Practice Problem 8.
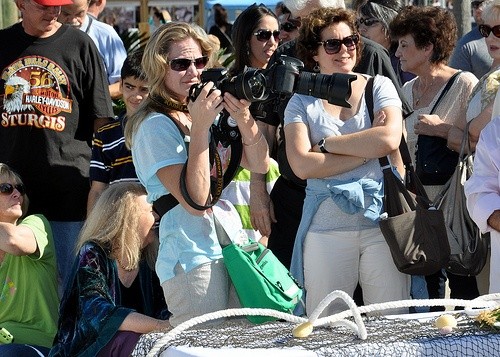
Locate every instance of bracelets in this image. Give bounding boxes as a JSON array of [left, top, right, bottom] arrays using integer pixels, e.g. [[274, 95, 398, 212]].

[[364, 158, 367, 165], [242, 133, 264, 148]]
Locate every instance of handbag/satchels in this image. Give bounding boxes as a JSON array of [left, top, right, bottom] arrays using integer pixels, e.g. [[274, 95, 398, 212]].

[[433, 160, 489, 276], [379, 171, 452, 275], [415, 70, 464, 186], [221, 242, 304, 325]]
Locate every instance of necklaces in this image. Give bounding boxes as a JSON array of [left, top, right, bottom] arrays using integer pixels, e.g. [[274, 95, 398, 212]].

[[415, 68, 441, 106], [117, 253, 136, 272]]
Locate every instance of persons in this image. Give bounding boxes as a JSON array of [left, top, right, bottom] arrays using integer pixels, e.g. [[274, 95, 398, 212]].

[[0, 162, 60, 357], [47, 182, 172, 357], [284, 8, 412, 320], [0, 0, 500, 313], [125, 23, 270, 327], [386, 5, 480, 312]]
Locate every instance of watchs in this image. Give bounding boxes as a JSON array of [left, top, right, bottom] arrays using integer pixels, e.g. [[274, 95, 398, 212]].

[[318, 137, 328, 153]]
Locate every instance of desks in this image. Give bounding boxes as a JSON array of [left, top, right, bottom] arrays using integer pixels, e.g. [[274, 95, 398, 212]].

[[130, 308, 500, 357]]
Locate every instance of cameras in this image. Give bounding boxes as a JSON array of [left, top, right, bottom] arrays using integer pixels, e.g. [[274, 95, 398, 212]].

[[194, 66, 268, 103], [267, 53, 358, 108]]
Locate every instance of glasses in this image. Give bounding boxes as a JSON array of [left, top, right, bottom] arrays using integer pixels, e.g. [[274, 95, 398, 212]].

[[287, 13, 301, 28], [471, 0, 484, 7], [169, 56, 210, 72], [0, 183, 25, 196], [254, 29, 280, 42], [280, 21, 294, 33], [320, 35, 359, 54], [478, 23, 500, 38], [360, 17, 380, 27]]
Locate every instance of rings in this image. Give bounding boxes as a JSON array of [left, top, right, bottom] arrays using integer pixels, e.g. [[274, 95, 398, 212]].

[[234, 109, 239, 114], [417, 119, 420, 125]]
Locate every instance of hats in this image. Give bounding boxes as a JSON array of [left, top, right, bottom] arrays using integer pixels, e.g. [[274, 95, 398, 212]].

[[32, 0, 74, 5], [154, 7, 171, 24]]
